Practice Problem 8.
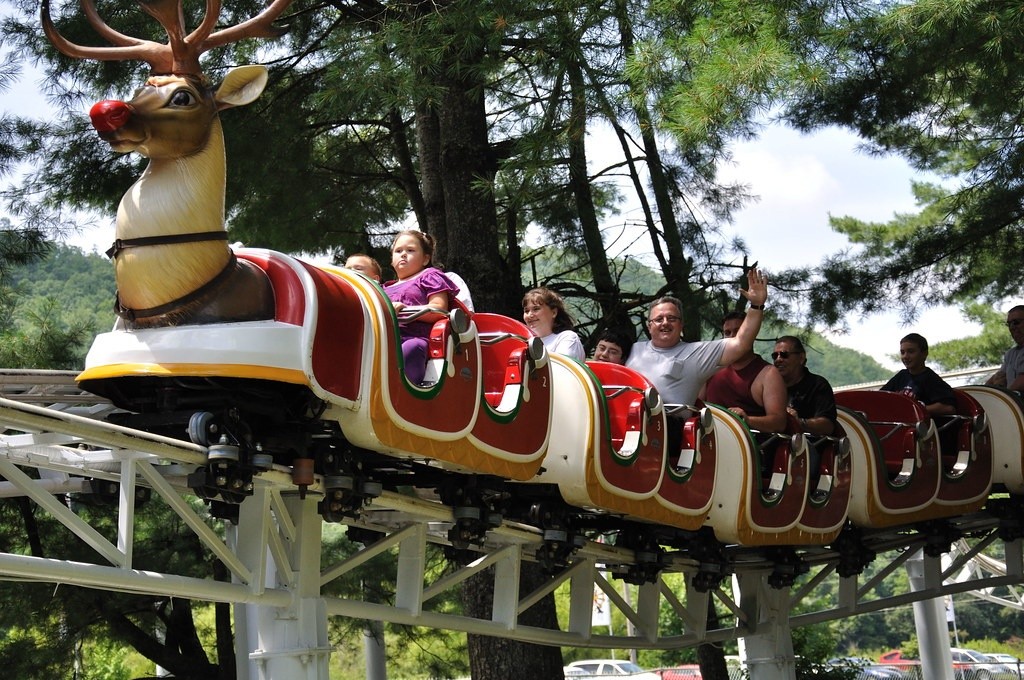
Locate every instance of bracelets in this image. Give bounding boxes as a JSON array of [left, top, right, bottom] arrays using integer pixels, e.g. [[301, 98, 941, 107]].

[[751, 304, 765, 310], [799, 418, 806, 425]]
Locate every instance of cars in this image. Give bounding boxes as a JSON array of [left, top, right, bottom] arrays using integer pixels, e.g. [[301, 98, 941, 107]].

[[564, 658, 661, 680], [655, 634, 1024, 680]]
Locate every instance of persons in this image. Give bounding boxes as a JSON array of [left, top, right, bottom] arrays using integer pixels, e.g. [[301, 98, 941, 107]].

[[985, 305, 1024, 406], [522, 288, 586, 363], [697, 310, 788, 476], [594, 329, 632, 364], [771, 335, 837, 480], [345, 253, 381, 282], [625, 268, 768, 454], [879, 333, 957, 456], [379, 230, 459, 385]]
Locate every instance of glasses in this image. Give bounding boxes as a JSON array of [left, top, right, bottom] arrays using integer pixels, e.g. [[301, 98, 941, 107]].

[[1006, 318, 1024, 326], [771, 350, 800, 359], [649, 314, 682, 323]]
